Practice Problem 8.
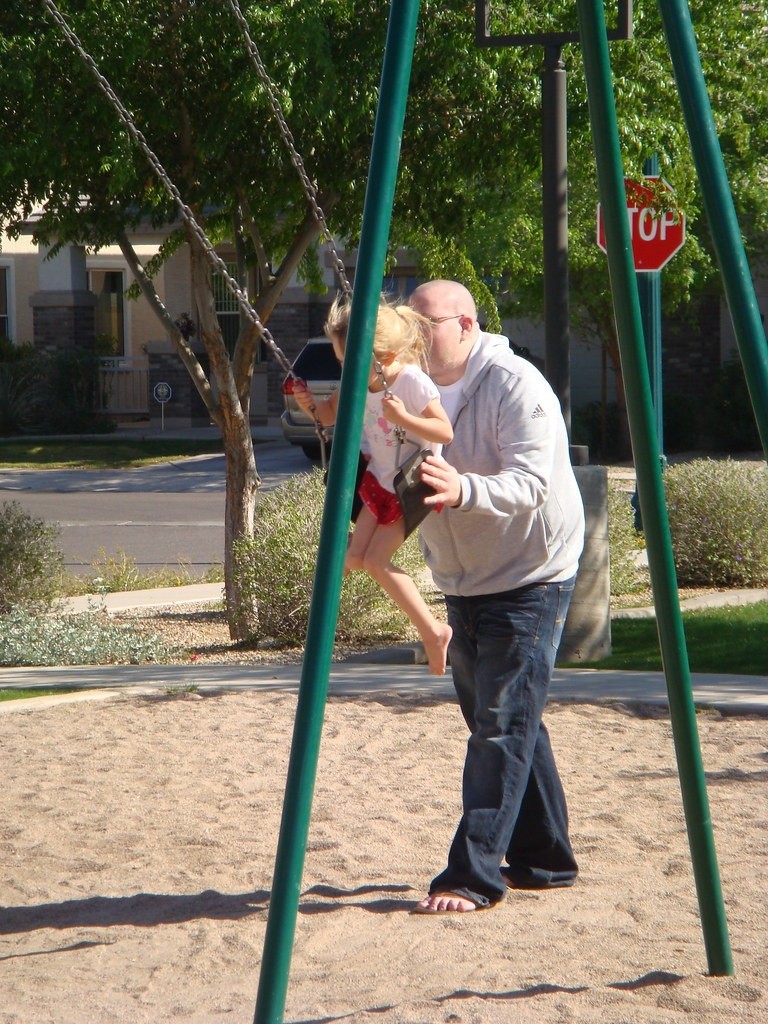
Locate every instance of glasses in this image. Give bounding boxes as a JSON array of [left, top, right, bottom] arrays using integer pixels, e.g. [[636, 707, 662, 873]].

[[418, 313, 464, 326]]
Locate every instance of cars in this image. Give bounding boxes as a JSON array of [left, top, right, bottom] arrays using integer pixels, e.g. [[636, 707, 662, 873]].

[[281, 335, 530, 459]]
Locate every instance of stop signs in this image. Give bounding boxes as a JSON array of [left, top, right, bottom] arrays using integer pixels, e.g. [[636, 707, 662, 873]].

[[595, 176, 685, 272]]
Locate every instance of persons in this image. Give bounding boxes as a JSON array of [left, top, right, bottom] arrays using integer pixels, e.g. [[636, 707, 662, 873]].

[[390, 276, 591, 916], [285, 297, 462, 677]]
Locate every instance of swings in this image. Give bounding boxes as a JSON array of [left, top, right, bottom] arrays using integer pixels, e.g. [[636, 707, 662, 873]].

[[39, 1, 440, 541]]
